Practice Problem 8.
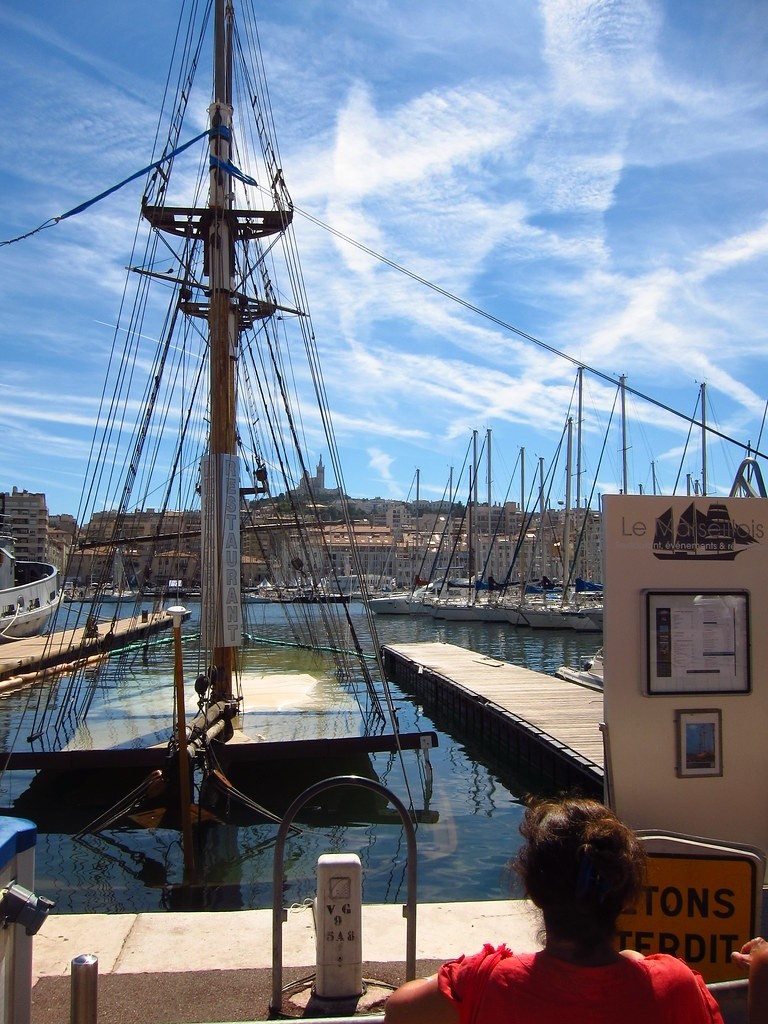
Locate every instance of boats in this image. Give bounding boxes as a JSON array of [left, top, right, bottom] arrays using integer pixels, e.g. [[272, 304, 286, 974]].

[[0, 508, 66, 646], [555, 647, 605, 693]]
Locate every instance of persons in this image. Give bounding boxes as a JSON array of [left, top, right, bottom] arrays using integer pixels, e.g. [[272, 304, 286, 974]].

[[80, 587, 84, 597], [515, 586, 519, 595], [384, 793, 768, 1024], [568, 588, 572, 601]]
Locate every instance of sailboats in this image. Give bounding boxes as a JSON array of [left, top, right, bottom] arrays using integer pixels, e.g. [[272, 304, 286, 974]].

[[57, 364, 768, 635]]
